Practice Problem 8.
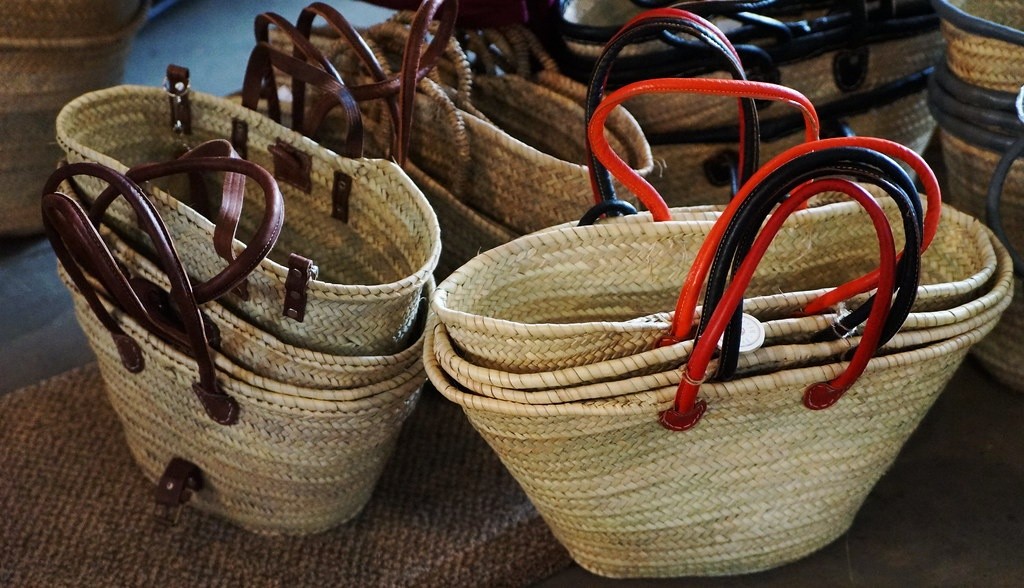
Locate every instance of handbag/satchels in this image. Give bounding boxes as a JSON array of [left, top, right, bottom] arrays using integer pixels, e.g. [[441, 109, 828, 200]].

[[38, 1, 1024, 579]]
